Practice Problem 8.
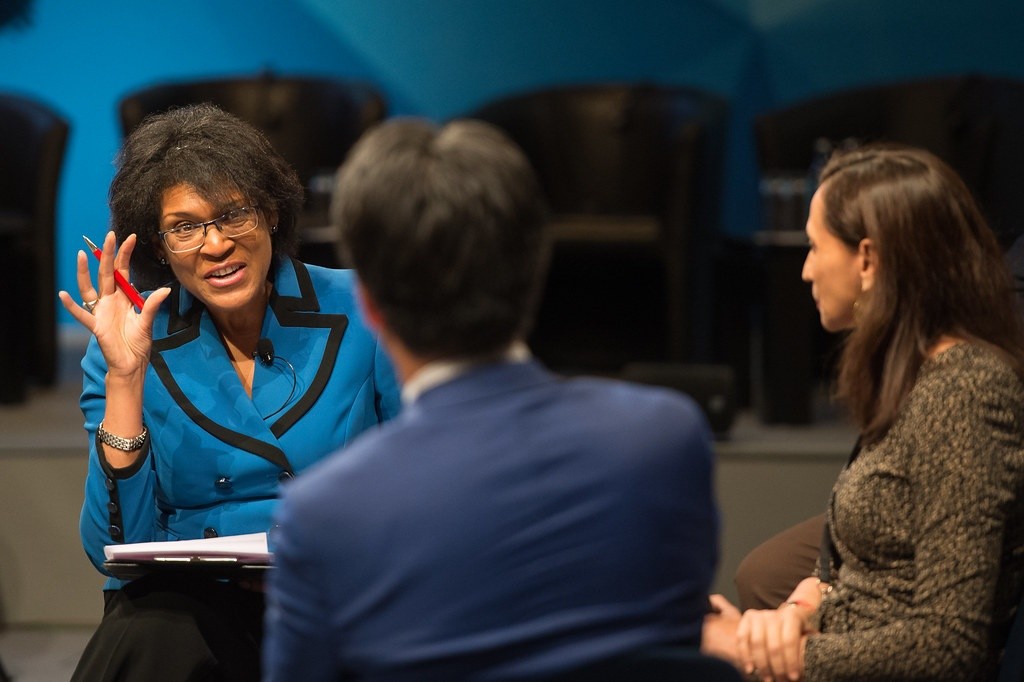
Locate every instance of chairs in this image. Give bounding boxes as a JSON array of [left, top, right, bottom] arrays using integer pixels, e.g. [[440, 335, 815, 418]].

[[0, 91, 71, 407], [751, 68, 1024, 420], [114, 72, 394, 269], [446, 75, 727, 376]]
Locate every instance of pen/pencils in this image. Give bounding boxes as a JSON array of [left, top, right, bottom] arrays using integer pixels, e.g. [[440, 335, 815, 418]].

[[82, 235, 145, 311]]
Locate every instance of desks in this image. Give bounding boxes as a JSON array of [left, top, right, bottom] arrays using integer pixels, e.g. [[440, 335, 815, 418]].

[[297, 215, 663, 337]]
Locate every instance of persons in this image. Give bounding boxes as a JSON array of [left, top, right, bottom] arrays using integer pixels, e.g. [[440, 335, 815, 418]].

[[264, 114, 722, 682], [696, 143, 1024, 682], [59, 107, 405, 681]]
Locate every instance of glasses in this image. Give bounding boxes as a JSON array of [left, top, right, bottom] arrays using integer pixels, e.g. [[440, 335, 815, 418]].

[[157, 202, 258, 253]]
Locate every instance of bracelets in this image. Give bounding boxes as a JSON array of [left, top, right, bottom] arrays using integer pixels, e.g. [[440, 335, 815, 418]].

[[99, 419, 147, 451]]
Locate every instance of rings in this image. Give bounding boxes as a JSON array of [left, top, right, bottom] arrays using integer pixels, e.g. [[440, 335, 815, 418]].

[[83, 301, 98, 310]]
